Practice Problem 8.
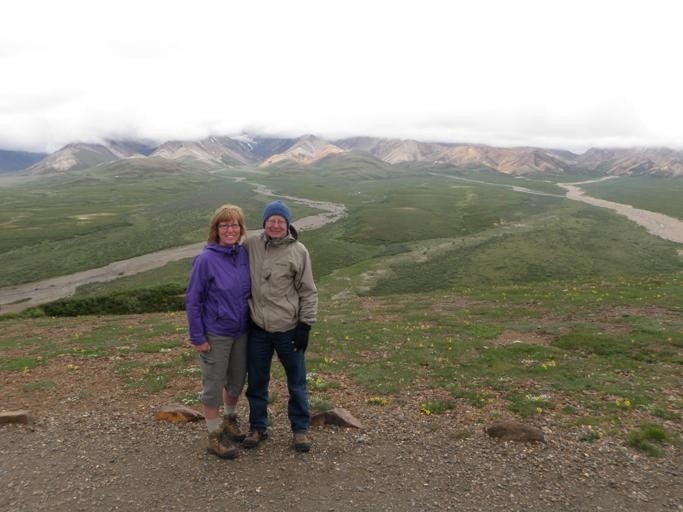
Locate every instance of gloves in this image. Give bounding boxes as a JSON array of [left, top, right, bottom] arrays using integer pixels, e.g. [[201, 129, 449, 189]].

[[291, 321, 311, 353]]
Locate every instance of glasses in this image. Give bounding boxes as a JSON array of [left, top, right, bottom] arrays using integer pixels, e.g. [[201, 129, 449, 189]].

[[265, 219, 288, 224], [216, 224, 239, 229]]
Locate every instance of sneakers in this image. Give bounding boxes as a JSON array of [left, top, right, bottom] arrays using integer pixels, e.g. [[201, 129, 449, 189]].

[[206, 430, 238, 458], [292, 433, 310, 451], [222, 413, 245, 441], [242, 431, 267, 447]]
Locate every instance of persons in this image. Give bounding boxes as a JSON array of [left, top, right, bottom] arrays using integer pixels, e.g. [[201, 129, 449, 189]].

[[185, 204, 252, 460], [240, 199, 318, 454]]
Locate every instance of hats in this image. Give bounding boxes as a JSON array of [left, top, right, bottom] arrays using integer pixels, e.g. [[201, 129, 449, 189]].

[[262, 200, 291, 228]]
